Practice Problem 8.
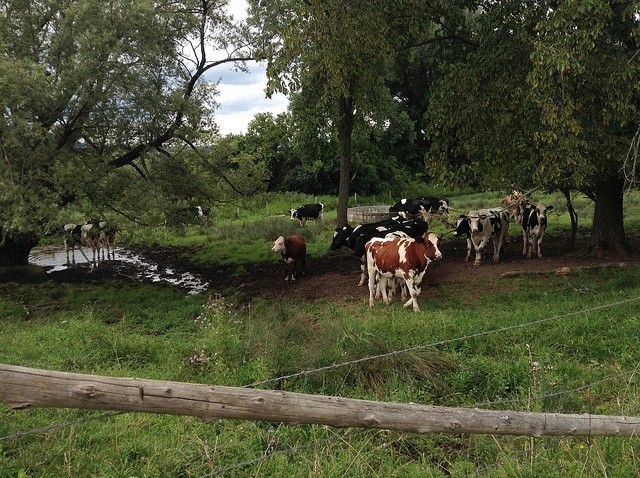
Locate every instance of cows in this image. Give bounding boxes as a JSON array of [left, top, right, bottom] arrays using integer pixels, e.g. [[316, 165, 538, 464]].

[[522, 203, 553, 259], [271, 235, 306, 280], [288, 203, 324, 226], [354, 220, 428, 287], [389, 198, 432, 222], [364, 232, 443, 312], [84, 220, 116, 260], [330, 224, 355, 251], [417, 197, 449, 216], [453, 214, 471, 261], [504, 190, 531, 225], [347, 220, 409, 246], [468, 208, 510, 265], [62, 224, 104, 264], [185, 206, 211, 225]]
